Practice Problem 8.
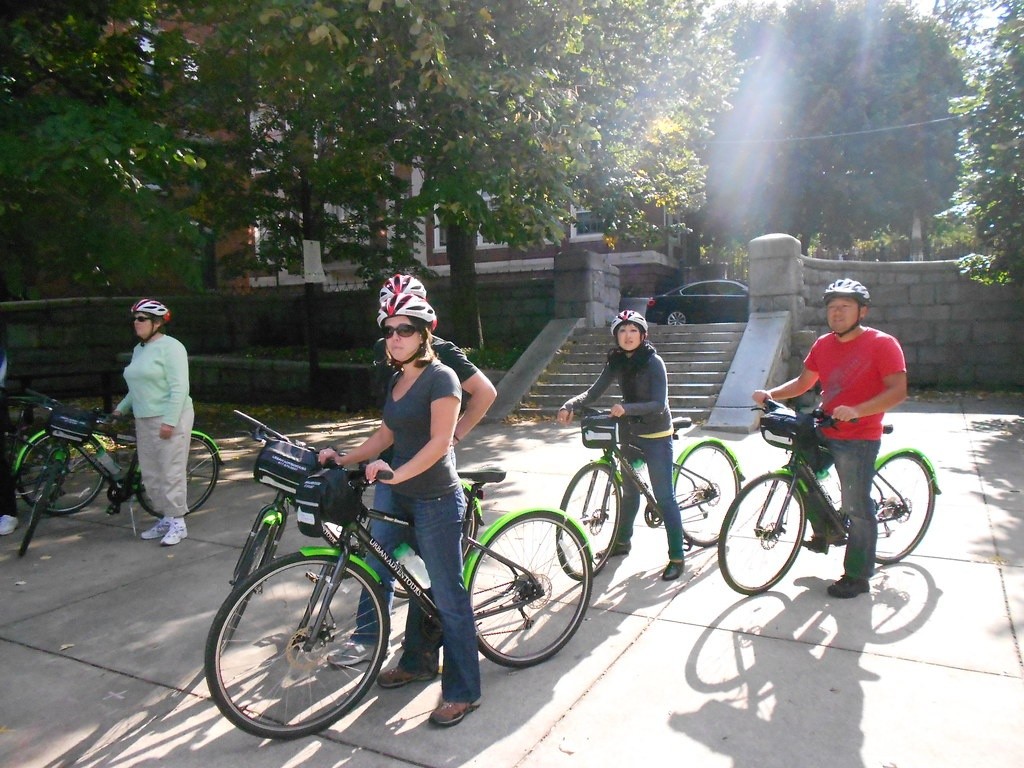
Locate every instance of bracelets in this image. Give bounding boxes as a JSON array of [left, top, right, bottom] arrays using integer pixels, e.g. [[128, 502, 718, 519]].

[[454, 435, 460, 442]]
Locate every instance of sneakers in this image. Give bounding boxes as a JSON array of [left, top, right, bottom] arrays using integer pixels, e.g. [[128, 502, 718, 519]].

[[325, 641, 393, 666], [160, 520, 188, 546], [428, 695, 481, 727], [376, 664, 437, 688], [0, 514, 20, 537], [140, 516, 173, 540]]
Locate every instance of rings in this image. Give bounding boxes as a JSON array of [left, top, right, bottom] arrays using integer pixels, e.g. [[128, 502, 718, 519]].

[[560, 418, 563, 420], [324, 452, 326, 454]]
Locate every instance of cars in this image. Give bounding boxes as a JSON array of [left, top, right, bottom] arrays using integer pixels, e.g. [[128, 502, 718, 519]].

[[644, 280, 750, 325]]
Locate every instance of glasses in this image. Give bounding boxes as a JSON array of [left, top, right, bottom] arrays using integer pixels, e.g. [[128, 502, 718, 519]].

[[130, 316, 153, 321], [380, 323, 419, 339]]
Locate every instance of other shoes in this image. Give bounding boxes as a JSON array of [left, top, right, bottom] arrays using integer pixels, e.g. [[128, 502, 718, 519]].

[[826, 576, 870, 599], [809, 536, 847, 551], [594, 541, 631, 558], [661, 558, 685, 582]]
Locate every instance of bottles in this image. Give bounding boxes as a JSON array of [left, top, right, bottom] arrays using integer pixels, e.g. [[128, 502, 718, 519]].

[[95, 447, 122, 474], [632, 458, 653, 489], [393, 543, 433, 590], [817, 469, 842, 503]]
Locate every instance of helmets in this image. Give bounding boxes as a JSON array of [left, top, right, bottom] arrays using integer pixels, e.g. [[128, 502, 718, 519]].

[[610, 309, 649, 335], [819, 278, 872, 309], [129, 298, 172, 323], [376, 273, 427, 301], [375, 292, 437, 334]]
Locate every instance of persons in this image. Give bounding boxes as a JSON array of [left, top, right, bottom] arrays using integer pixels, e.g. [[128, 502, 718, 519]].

[[557, 310, 685, 581], [752, 278, 907, 598], [0, 347, 19, 535], [318, 294, 481, 726], [100, 299, 195, 545], [326, 274, 498, 666]]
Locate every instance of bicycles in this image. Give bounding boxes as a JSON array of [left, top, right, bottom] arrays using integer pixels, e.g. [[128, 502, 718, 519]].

[[556, 407, 746, 581], [6, 389, 225, 557], [205, 411, 593, 739], [719, 399, 942, 596]]
